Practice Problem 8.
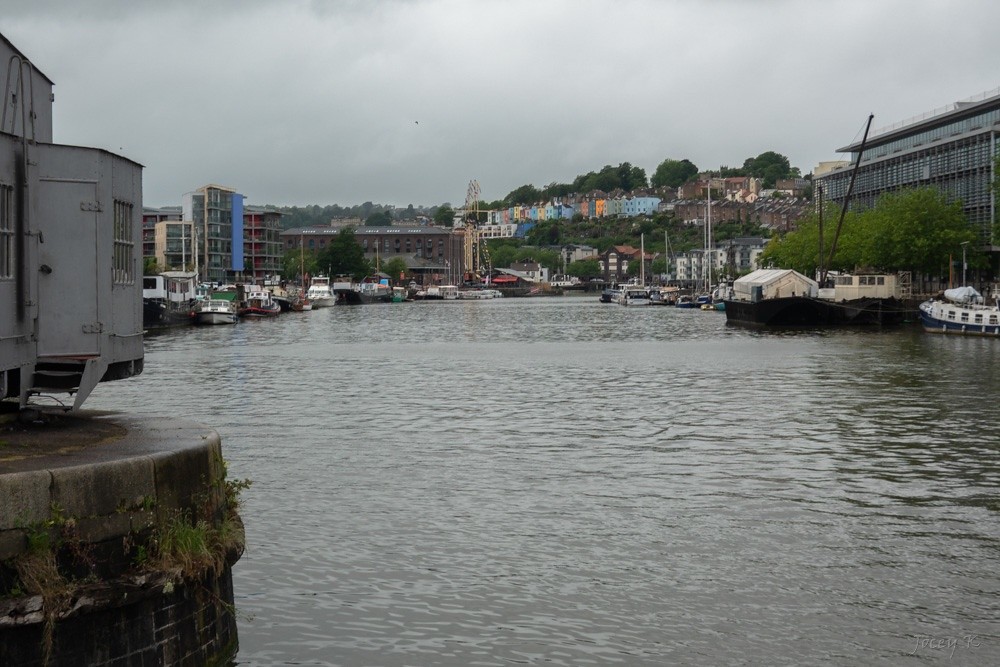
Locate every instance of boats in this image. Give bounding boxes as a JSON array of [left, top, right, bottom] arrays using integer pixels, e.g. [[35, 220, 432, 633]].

[[599, 184, 733, 310], [142, 270, 203, 329], [918, 253, 1000, 337], [196, 299, 238, 325], [242, 291, 281, 317], [334, 281, 392, 304], [415, 285, 504, 301], [391, 286, 409, 302], [307, 275, 337, 306], [262, 285, 291, 313]]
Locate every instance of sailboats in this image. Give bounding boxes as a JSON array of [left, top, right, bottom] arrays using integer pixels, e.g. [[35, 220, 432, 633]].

[[290, 230, 315, 311]]
